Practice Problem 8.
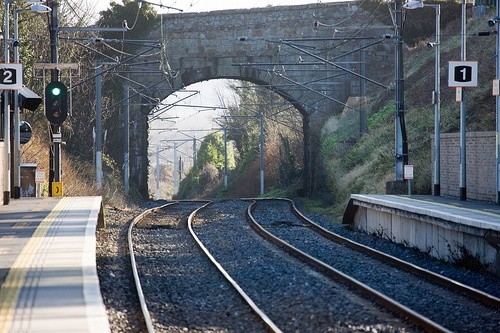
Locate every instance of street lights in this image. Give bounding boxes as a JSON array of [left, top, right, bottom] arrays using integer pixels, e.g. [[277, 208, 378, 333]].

[[402, 0, 441, 196], [14, 5, 52, 199]]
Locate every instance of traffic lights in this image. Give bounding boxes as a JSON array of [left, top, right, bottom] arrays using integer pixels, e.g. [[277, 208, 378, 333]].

[[45, 82, 68, 125]]
